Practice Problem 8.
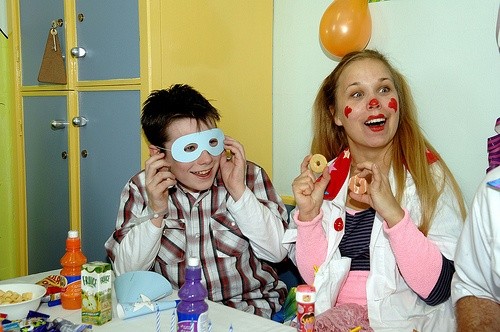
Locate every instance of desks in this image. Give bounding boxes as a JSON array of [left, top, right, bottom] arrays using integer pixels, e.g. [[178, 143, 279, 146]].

[[0, 268, 296, 332]]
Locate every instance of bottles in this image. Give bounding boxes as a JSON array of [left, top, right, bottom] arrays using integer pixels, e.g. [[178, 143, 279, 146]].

[[60, 231, 88, 309], [296, 285, 315, 332], [176, 257, 210, 332]]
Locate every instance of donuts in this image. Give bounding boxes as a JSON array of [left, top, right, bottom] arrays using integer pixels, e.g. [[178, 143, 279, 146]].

[[348, 175, 368, 195], [310, 153, 327, 173]]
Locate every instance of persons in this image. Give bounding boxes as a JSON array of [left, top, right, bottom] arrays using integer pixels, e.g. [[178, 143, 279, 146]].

[[104, 84, 288, 320], [283, 50, 469, 332], [450, 167, 500, 332]]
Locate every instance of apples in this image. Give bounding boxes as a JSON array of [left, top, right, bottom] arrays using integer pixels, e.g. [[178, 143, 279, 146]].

[[82, 296, 97, 310]]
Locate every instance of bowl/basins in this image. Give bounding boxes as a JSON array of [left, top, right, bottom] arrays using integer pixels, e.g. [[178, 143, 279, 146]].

[[0, 283, 47, 320]]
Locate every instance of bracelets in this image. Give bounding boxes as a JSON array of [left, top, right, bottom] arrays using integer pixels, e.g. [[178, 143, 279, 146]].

[[130, 207, 168, 223]]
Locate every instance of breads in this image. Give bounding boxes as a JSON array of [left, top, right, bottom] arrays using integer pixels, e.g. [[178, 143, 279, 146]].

[[0, 290, 33, 305]]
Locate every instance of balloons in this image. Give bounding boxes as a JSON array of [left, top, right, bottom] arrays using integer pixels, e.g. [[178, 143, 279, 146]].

[[319, 0, 372, 57]]
